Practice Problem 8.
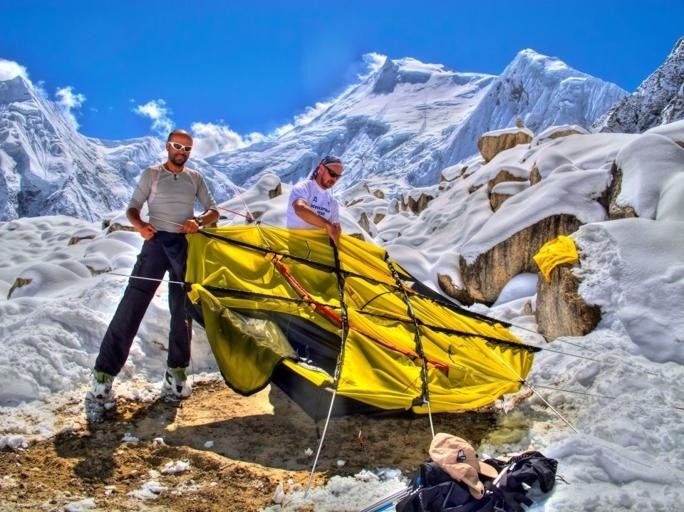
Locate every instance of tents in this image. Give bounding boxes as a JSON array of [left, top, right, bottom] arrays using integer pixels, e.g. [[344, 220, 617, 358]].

[[183, 210, 542, 426]]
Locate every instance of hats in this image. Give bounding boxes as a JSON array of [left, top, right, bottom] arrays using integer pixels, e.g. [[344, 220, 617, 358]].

[[429, 432, 499, 478]]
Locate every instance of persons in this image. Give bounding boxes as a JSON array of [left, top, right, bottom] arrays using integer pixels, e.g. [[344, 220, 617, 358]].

[[89, 128, 220, 400], [286, 156, 343, 247]]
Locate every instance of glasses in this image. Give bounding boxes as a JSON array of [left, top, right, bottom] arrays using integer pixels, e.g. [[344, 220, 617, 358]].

[[324, 164, 341, 180], [168, 141, 192, 152]]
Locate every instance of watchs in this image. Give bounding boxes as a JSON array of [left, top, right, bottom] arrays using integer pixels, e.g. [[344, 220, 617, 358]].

[[192, 213, 205, 228]]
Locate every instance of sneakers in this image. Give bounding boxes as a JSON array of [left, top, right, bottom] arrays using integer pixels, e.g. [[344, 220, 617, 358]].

[[164, 365, 192, 399], [92, 373, 112, 399]]
